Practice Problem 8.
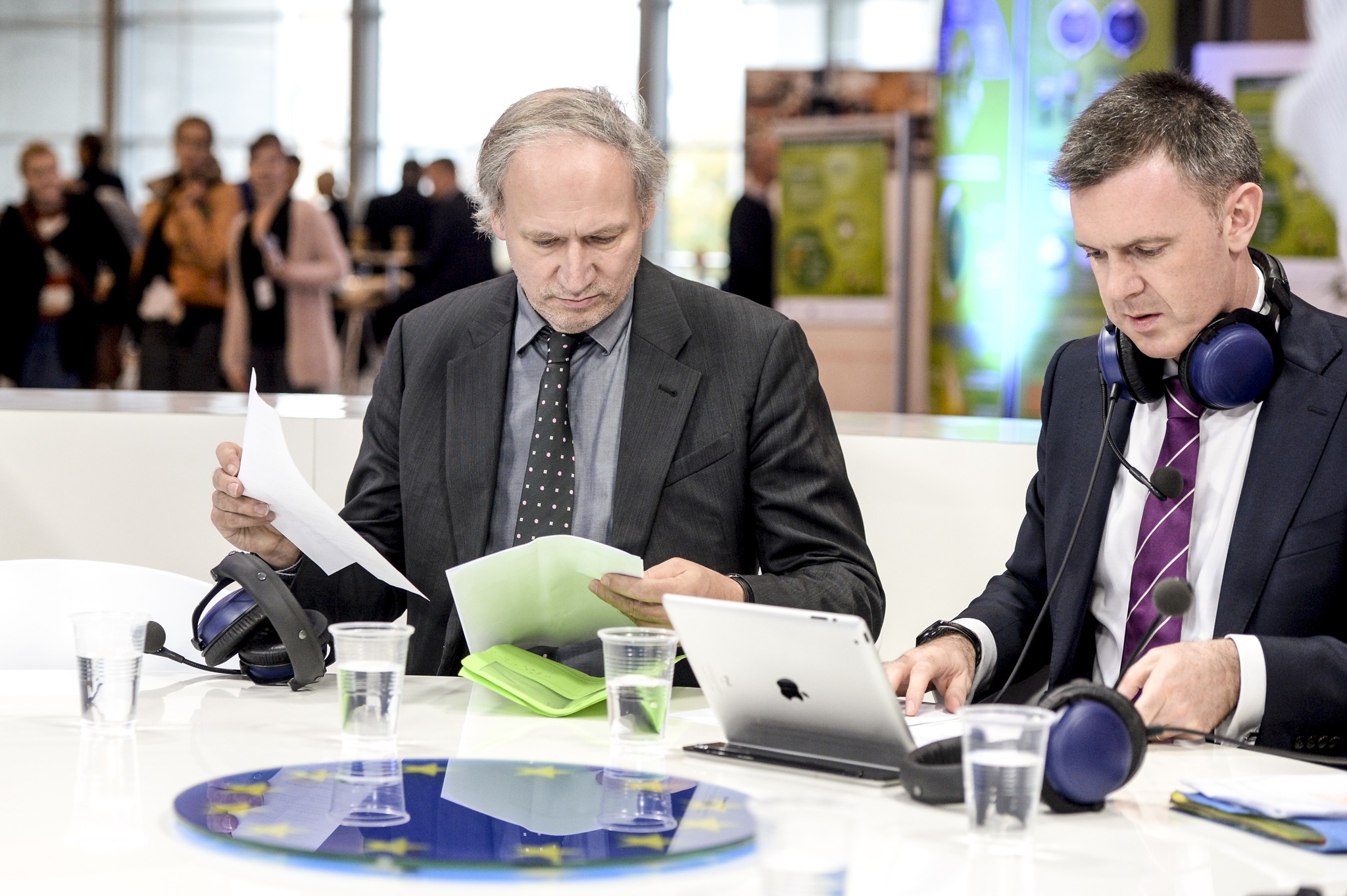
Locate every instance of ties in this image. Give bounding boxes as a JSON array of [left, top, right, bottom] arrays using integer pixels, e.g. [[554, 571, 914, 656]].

[[1116, 368, 1208, 743], [512, 326, 596, 660]]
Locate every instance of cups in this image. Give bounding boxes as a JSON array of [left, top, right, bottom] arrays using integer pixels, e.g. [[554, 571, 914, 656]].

[[326, 620, 416, 741], [69, 606, 150, 726], [596, 769, 679, 833], [598, 626, 679, 741], [334, 760, 410, 826], [957, 704, 1060, 835]]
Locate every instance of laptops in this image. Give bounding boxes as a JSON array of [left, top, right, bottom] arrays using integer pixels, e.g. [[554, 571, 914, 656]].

[[661, 593, 1027, 767]]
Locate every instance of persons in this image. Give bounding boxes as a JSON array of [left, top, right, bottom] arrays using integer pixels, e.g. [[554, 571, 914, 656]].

[[208, 86, 886, 687], [716, 130, 784, 310], [0, 115, 508, 397], [880, 62, 1347, 759]]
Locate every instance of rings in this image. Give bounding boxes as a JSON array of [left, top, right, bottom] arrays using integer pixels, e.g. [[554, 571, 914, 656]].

[[1153, 732, 1162, 743]]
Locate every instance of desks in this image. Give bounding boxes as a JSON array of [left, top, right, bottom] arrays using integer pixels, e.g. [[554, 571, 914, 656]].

[[0, 676, 1347, 896]]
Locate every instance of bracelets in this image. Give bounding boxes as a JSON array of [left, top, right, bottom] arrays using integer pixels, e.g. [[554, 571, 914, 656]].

[[916, 618, 982, 666], [722, 571, 757, 603]]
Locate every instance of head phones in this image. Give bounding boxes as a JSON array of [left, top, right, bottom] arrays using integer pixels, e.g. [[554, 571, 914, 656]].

[[187, 551, 335, 690], [898, 680, 1148, 815], [1100, 247, 1291, 409]]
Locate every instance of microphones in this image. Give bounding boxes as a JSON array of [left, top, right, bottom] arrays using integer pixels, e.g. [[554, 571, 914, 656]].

[[1114, 577, 1194, 693], [1103, 384, 1187, 500], [134, 620, 246, 676]]
